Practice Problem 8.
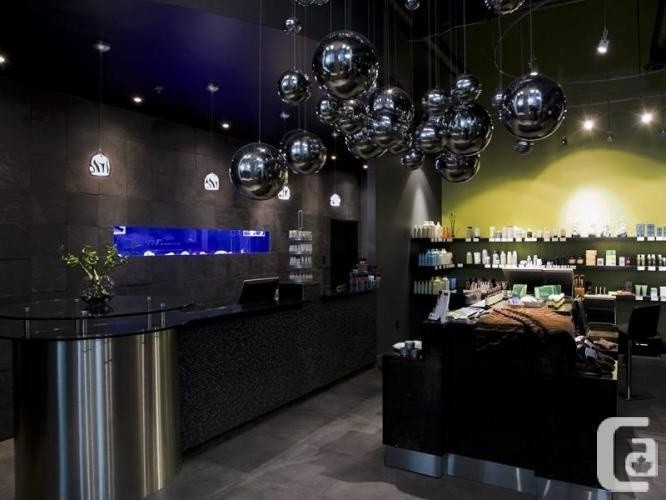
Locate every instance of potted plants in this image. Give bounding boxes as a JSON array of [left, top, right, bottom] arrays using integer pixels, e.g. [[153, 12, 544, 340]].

[[58, 242, 132, 309]]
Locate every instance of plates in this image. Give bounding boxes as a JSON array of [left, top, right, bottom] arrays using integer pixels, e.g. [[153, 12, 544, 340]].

[[393, 340, 423, 351]]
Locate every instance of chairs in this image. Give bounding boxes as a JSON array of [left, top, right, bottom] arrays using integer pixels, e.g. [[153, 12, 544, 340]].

[[571, 298, 621, 363], [613, 303, 661, 400]]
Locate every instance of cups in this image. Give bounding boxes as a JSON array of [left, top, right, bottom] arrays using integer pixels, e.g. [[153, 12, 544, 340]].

[[399, 341, 420, 360]]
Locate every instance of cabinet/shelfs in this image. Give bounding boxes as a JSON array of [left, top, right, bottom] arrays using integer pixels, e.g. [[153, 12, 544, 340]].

[[407, 234, 457, 329], [287, 209, 313, 281], [455, 237, 665, 307]]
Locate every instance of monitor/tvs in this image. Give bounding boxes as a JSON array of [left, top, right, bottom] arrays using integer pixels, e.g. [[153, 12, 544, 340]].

[[573, 294, 590, 338], [237, 277, 279, 305]]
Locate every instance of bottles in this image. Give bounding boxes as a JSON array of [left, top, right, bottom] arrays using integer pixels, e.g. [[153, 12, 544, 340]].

[[411, 219, 457, 296], [465, 248, 517, 265], [287, 229, 314, 282], [626, 257, 631, 265], [366, 274, 375, 289], [568, 255, 585, 265], [466, 225, 480, 239]]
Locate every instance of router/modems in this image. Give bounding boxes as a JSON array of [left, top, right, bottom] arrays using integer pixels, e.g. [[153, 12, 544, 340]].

[[584, 286, 616, 300]]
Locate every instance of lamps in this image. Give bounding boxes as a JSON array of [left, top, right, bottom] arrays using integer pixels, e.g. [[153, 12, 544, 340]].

[[87, 42, 115, 178], [202, 82, 223, 194]]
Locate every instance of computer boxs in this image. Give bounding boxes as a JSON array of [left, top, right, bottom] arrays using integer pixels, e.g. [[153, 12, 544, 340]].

[[583, 299, 616, 325]]
